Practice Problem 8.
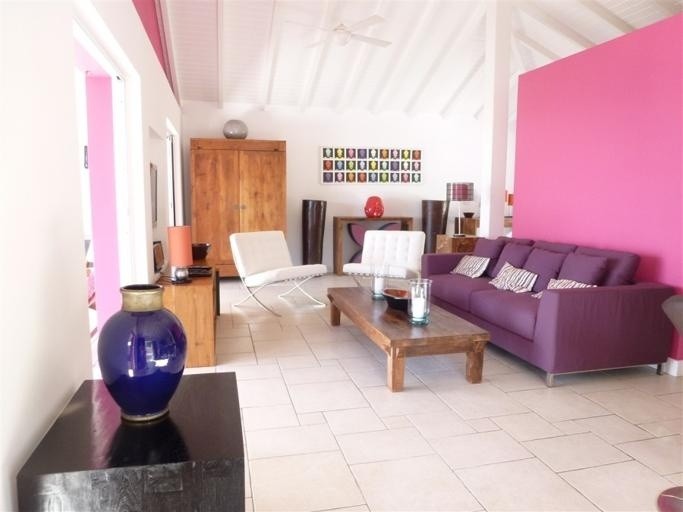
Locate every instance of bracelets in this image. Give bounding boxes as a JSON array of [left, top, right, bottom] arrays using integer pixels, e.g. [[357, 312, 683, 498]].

[[190, 241, 211, 261], [463, 211, 475, 218]]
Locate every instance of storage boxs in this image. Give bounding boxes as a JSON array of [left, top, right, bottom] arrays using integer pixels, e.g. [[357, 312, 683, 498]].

[[226, 228, 329, 313], [342, 229, 427, 289]]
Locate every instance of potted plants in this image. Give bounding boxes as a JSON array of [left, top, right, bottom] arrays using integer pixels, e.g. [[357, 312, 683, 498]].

[[148, 162, 158, 230], [152, 239, 165, 272]]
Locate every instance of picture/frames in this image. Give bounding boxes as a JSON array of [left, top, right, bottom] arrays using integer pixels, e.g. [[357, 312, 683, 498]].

[[17, 369, 246, 511], [153, 267, 220, 367], [333, 215, 413, 275]]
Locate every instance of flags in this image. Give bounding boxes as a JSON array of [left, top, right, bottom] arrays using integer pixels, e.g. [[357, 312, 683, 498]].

[[95, 284, 188, 427]]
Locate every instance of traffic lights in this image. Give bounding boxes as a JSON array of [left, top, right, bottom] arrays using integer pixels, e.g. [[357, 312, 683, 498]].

[[491, 241, 534, 284], [556, 252, 608, 289], [519, 246, 565, 293], [471, 236, 504, 277]]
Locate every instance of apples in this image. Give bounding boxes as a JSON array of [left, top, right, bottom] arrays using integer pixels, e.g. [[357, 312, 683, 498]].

[[412, 297, 424, 319], [375, 277, 382, 293]]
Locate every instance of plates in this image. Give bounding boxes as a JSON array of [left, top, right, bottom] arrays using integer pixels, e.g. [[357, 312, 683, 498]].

[[188, 137, 287, 278], [453, 213, 512, 237], [435, 233, 484, 256]]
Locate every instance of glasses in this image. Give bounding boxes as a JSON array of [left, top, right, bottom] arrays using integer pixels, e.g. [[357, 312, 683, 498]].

[[445, 181, 475, 237], [165, 224, 194, 286]]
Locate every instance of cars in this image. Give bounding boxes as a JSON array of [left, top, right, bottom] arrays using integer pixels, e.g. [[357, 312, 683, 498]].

[[408, 278, 433, 327]]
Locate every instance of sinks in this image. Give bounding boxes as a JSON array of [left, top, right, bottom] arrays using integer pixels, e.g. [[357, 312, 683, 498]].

[[420, 235, 680, 387]]
